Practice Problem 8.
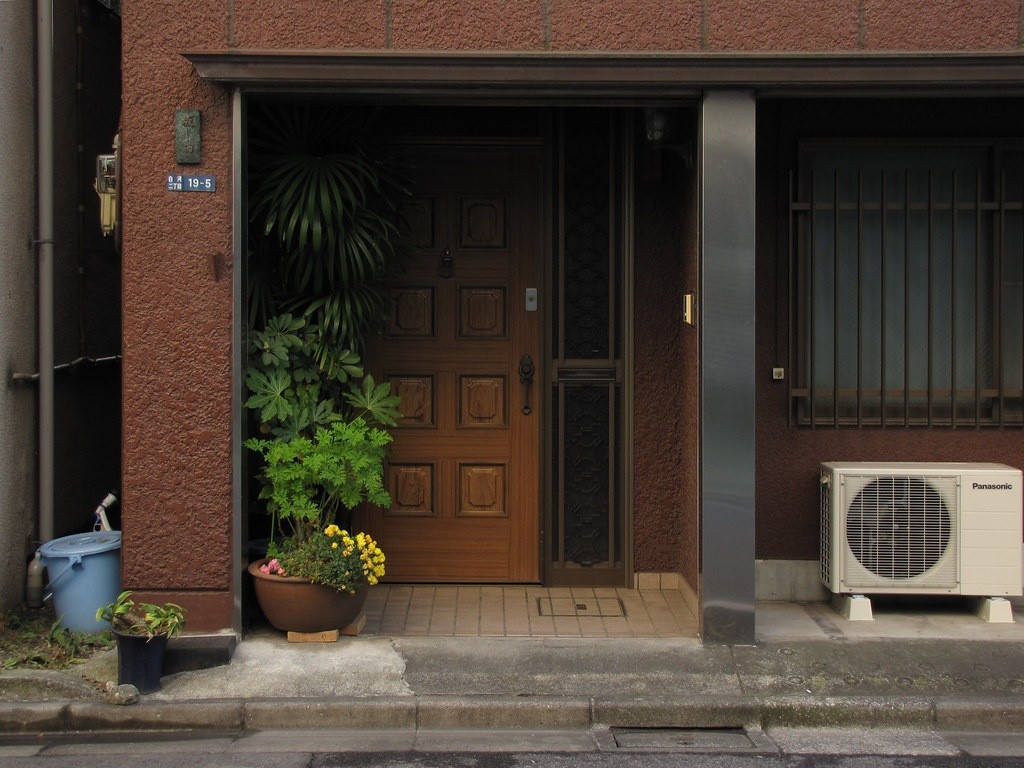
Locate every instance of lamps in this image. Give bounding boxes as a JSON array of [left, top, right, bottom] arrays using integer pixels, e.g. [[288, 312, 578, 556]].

[[640, 107, 693, 171]]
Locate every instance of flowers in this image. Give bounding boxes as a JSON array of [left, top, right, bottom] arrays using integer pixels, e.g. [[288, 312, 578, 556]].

[[259, 524, 387, 594]]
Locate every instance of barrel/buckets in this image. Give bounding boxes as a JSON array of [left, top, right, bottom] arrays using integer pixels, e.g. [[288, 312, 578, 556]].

[[38, 532, 122, 633]]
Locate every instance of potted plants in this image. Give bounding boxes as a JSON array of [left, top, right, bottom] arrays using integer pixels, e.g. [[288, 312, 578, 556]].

[[243, 98, 422, 633], [96, 591, 186, 695]]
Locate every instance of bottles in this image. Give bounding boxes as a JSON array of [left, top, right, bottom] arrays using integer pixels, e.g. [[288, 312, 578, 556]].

[[27, 551, 43, 608]]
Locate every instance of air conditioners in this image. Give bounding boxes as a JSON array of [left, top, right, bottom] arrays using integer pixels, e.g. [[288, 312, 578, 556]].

[[820, 460, 1024, 597]]
